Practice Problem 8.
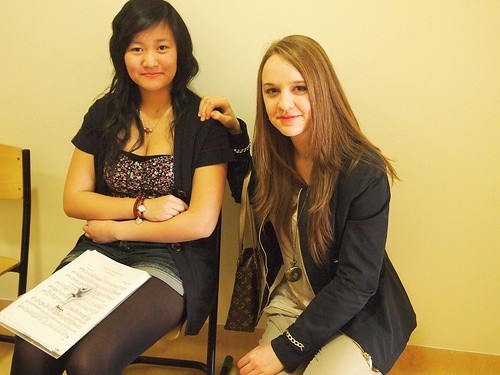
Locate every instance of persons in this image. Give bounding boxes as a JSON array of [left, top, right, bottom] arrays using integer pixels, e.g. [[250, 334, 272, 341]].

[[198, 34, 417, 375], [10, 0, 232, 375]]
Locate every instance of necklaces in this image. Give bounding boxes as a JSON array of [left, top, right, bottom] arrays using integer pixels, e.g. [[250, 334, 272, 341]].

[[138, 104, 171, 135], [287, 223, 302, 282]]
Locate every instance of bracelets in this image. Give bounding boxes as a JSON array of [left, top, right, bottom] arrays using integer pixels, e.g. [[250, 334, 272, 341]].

[[136, 197, 145, 225], [133, 197, 141, 218]]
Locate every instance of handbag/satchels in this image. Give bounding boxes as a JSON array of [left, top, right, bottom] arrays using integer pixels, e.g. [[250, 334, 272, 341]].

[[225, 171, 262, 332]]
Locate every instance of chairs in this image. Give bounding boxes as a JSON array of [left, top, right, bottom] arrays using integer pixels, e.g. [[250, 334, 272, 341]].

[[128, 206, 222, 375], [0, 144, 31, 344]]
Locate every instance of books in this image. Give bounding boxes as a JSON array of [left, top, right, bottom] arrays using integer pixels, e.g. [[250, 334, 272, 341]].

[[0, 250, 152, 359]]
